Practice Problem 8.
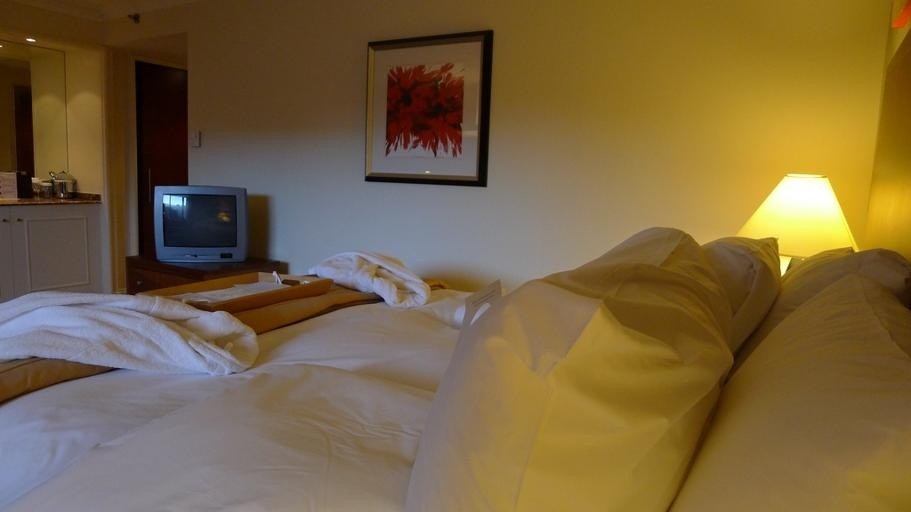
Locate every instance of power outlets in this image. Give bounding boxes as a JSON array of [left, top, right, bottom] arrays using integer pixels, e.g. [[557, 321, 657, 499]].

[[190, 130, 201, 146]]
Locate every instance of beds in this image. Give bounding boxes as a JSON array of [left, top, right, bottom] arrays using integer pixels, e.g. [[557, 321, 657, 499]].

[[1, 272, 911, 511]]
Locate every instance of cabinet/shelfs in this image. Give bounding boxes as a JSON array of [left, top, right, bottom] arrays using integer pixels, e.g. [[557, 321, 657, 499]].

[[127, 256, 288, 299], [0, 193, 103, 301]]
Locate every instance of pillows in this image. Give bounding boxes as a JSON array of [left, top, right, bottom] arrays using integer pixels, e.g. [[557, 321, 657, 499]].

[[403, 227, 737, 511], [702, 237, 779, 351], [748, 247, 911, 352], [669, 265, 909, 512]]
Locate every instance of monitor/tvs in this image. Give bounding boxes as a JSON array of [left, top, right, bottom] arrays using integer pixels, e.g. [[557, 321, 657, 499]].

[[155, 186, 248, 269]]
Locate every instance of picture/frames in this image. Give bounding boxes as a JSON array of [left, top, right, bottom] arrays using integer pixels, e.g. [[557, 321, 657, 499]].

[[364, 29, 493, 188]]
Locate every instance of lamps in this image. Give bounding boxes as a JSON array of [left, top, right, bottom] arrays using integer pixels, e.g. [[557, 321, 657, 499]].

[[735, 174, 861, 272]]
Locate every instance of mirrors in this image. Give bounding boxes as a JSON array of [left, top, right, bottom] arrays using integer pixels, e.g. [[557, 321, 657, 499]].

[[0, 40, 68, 198]]
